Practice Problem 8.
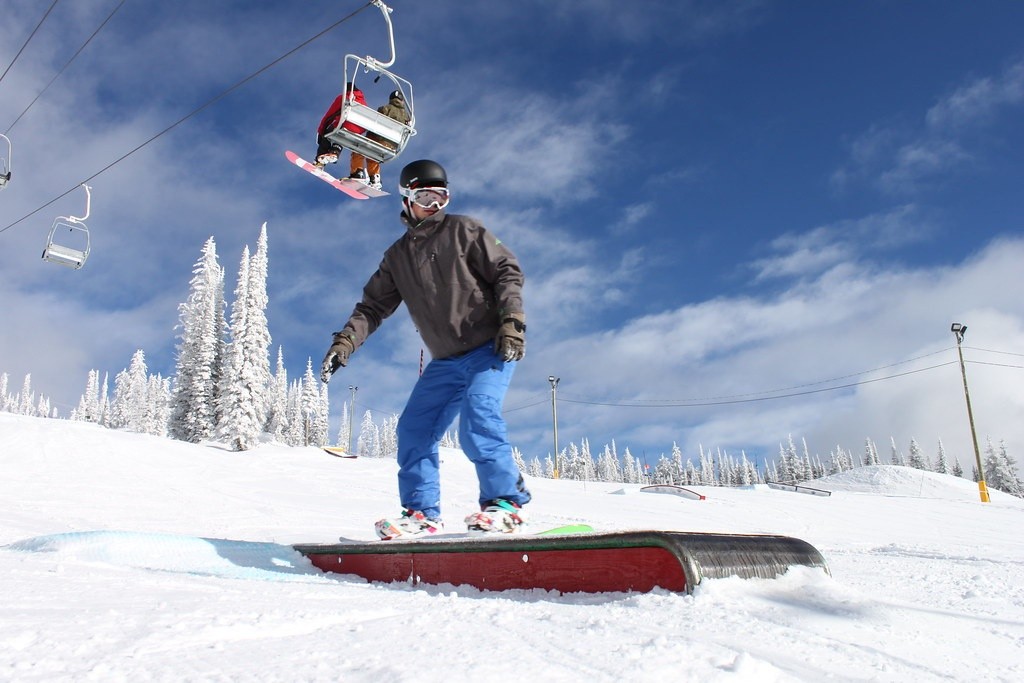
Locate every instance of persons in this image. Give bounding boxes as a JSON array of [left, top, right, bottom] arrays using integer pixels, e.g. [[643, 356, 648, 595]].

[[312, 81, 410, 191], [320, 160, 532, 541]]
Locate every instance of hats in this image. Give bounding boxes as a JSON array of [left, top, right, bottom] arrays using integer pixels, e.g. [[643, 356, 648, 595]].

[[389, 90, 404, 100], [346, 82, 357, 91]]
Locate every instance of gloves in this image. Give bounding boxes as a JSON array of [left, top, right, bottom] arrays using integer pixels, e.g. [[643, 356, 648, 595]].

[[320, 336, 353, 383], [318, 134, 321, 145], [494, 317, 526, 363]]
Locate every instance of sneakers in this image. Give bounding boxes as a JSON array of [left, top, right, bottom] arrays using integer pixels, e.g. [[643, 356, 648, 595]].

[[377, 512, 443, 537], [468, 501, 524, 532]]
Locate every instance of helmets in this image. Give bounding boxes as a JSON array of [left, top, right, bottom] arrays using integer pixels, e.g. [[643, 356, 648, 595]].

[[400, 159, 447, 188]]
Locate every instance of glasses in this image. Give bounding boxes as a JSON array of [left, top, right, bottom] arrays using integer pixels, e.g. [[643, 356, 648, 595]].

[[410, 187, 450, 210]]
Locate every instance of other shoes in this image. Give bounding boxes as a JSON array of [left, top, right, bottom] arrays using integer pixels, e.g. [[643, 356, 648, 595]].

[[312, 160, 324, 170], [316, 150, 339, 165], [367, 174, 382, 188], [348, 169, 366, 181]]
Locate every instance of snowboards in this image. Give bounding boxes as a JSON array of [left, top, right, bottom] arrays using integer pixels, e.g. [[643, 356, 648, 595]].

[[338, 524, 597, 546], [284, 150, 369, 201], [339, 177, 392, 198]]
